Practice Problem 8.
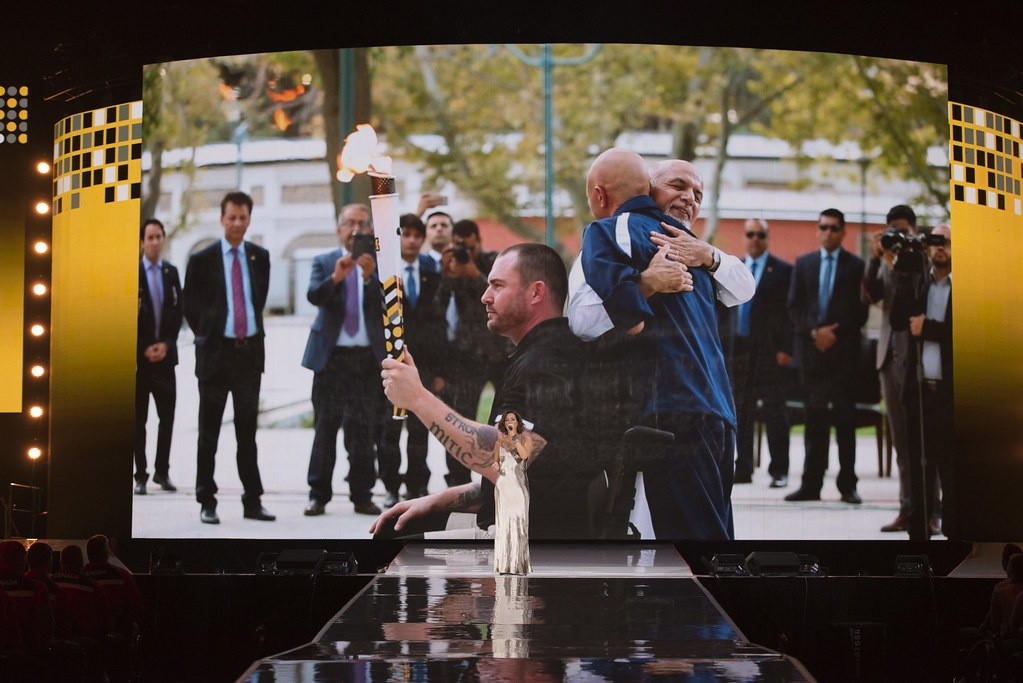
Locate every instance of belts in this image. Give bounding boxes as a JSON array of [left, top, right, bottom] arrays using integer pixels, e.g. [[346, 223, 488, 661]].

[[927, 380, 946, 390], [224, 336, 263, 348]]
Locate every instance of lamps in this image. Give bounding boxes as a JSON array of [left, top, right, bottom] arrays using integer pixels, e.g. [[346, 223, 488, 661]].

[[707, 551, 821, 578], [895, 555, 932, 576], [256, 548, 359, 576]]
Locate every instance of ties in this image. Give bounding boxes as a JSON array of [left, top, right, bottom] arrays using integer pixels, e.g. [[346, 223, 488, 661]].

[[819, 256, 835, 322], [405, 267, 417, 311], [345, 262, 360, 336], [740, 260, 758, 338], [230, 247, 248, 340], [150, 265, 161, 335]]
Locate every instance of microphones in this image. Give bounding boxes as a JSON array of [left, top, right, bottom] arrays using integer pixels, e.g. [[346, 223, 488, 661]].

[[509, 425, 513, 431]]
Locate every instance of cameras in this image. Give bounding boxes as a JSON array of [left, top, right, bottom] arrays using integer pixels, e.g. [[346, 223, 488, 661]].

[[452, 244, 470, 264], [879, 228, 906, 249]]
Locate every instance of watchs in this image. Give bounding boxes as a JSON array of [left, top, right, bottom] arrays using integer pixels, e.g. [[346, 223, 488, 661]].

[[707, 246, 720, 271]]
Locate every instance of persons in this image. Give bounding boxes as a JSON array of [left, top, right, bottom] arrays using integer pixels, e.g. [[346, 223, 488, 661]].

[[84, 534, 140, 596], [862, 204, 952, 532], [184, 193, 277, 524], [566, 148, 758, 539], [302, 203, 384, 512], [0, 541, 119, 635], [730, 218, 793, 488], [369, 243, 618, 537], [383, 194, 502, 512], [978, 543, 1023, 638], [493, 411, 533, 576], [784, 209, 869, 503], [135, 218, 183, 495]]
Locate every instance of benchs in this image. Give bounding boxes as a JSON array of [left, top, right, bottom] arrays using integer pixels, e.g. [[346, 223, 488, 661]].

[[753, 336, 893, 477]]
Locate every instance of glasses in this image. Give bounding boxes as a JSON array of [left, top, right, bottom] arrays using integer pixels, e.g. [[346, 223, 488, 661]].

[[743, 229, 767, 240], [820, 224, 845, 233]]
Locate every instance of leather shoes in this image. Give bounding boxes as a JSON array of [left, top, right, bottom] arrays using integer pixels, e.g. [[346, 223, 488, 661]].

[[153, 472, 176, 489], [731, 475, 751, 483], [304, 500, 326, 515], [882, 516, 910, 530], [353, 502, 381, 514], [244, 505, 276, 520], [134, 483, 146, 494], [201, 504, 220, 523], [929, 518, 941, 535], [770, 476, 788, 487]]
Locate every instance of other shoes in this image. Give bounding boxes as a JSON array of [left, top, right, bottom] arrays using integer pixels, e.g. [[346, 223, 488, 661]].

[[784, 489, 821, 501], [384, 497, 398, 506], [843, 489, 860, 502]]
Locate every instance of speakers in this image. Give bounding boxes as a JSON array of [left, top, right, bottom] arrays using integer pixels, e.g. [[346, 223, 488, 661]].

[[276, 548, 329, 576], [743, 552, 800, 575]]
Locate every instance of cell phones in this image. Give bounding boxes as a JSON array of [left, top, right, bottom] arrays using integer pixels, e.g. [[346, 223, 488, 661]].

[[924, 233, 946, 247], [352, 234, 376, 259]]
